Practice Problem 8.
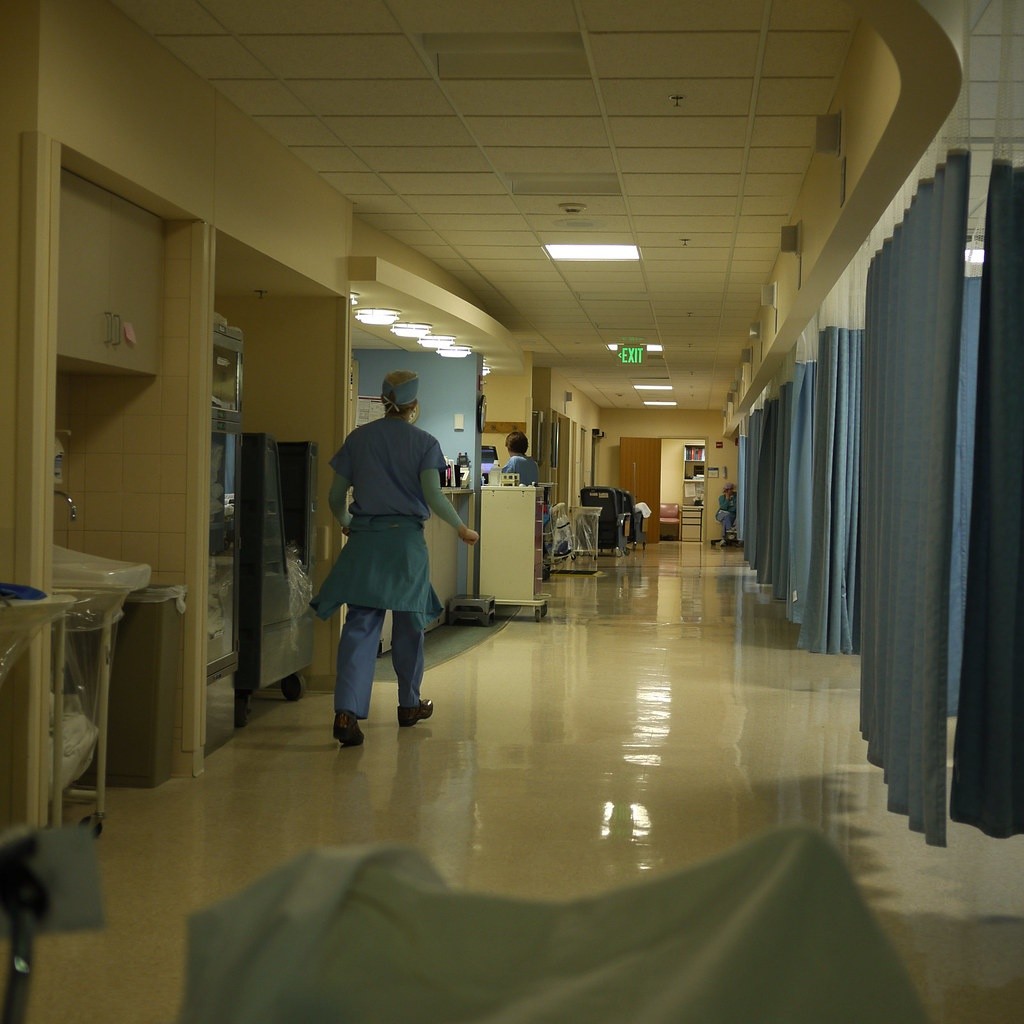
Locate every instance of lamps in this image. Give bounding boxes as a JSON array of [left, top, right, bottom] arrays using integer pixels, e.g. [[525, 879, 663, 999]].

[[482, 366, 491, 377], [966, 234, 986, 264], [417, 335, 456, 349], [350, 291, 359, 305], [353, 308, 402, 325], [434, 345, 472, 358], [390, 323, 432, 338]]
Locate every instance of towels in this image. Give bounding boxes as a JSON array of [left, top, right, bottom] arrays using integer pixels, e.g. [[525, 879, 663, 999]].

[[633, 501, 653, 519]]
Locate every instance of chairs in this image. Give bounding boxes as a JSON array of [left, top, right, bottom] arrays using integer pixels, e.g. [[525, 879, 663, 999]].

[[660, 503, 682, 541], [580, 486, 646, 556]]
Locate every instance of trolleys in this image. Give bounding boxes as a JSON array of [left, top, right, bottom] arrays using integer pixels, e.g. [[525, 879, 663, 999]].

[[580, 487, 629, 557], [620, 492, 648, 550]]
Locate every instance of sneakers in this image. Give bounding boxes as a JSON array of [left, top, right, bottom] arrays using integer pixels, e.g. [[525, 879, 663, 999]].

[[333, 712, 364, 746], [398, 699, 434, 727]]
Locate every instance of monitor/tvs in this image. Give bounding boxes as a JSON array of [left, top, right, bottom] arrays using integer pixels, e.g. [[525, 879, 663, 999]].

[[480, 446, 498, 486]]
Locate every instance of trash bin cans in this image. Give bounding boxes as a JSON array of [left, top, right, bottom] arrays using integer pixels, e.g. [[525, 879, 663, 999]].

[[77, 579, 189, 793]]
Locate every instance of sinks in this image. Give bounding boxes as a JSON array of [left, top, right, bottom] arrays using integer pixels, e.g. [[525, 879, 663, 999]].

[[54, 538, 157, 630]]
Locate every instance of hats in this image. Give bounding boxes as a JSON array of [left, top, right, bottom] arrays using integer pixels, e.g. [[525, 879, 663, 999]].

[[382, 369, 418, 405]]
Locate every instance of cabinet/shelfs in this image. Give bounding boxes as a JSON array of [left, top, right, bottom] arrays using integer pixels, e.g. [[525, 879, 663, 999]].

[[479, 486, 552, 623], [200, 321, 237, 759], [681, 506, 701, 542], [683, 441, 704, 508]]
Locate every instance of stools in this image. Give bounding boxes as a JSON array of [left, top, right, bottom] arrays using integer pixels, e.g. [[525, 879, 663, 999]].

[[715, 517, 741, 547]]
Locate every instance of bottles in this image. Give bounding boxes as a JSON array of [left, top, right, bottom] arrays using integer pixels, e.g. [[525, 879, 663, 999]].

[[489, 459, 501, 485]]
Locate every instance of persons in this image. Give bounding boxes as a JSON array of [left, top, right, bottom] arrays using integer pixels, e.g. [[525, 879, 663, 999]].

[[500, 431, 539, 488], [309, 369, 480, 747], [715, 483, 737, 547]]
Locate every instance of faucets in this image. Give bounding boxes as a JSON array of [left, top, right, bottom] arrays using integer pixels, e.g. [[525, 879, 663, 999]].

[[55, 486, 81, 523]]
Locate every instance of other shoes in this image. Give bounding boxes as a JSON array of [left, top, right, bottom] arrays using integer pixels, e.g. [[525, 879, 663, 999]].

[[727, 534, 735, 540], [720, 540, 727, 546]]
